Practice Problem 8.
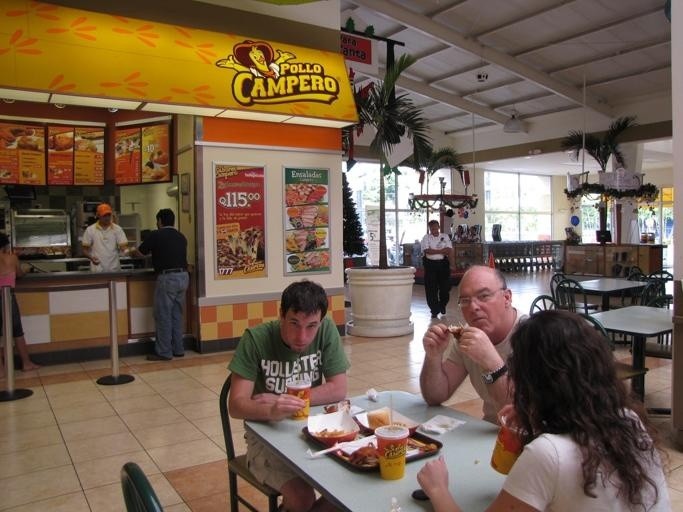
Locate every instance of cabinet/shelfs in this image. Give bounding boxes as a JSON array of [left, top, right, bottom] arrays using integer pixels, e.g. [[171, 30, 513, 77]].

[[449, 242, 484, 279], [117, 213, 142, 249], [17, 254, 153, 274]]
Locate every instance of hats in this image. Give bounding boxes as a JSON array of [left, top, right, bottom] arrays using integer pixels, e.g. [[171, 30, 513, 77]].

[[96, 204, 113, 217]]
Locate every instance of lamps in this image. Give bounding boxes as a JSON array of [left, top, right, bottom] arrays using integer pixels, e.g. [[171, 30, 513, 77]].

[[502, 102, 528, 135]]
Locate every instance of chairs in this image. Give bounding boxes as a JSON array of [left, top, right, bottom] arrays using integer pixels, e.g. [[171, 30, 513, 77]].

[[216, 370, 284, 511], [117, 460, 168, 511], [528, 266, 680, 429]]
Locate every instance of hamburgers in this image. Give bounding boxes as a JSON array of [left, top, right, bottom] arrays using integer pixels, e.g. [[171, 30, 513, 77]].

[[350, 446, 377, 467]]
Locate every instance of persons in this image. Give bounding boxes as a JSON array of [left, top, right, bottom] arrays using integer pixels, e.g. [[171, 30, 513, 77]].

[[0, 231, 39, 371], [419, 264, 527, 425], [129, 207, 191, 360], [82, 202, 128, 273], [419, 219, 453, 318], [226, 280, 351, 511], [414, 309, 671, 512]]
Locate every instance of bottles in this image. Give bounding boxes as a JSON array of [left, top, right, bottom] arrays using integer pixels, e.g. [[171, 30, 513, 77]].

[[640, 232, 656, 244], [389, 495, 402, 512]]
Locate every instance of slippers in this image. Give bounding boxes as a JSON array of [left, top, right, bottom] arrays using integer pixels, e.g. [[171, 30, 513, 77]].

[[20, 363, 42, 372]]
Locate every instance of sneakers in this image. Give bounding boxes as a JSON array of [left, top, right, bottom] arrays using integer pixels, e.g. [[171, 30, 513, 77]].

[[146, 353, 172, 361], [173, 353, 185, 357], [429, 305, 446, 319]]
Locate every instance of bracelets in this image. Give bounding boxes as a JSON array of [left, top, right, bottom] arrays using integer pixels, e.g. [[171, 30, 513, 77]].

[[480, 363, 507, 385]]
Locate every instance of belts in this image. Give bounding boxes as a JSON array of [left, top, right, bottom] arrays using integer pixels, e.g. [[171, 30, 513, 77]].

[[157, 267, 189, 275]]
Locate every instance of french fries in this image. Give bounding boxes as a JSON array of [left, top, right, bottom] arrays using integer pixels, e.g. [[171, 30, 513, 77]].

[[316, 428, 348, 438]]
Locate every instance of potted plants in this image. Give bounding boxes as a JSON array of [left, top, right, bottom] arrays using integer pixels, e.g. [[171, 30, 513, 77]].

[[407, 146, 480, 216], [344, 50, 435, 340], [561, 114, 657, 203]]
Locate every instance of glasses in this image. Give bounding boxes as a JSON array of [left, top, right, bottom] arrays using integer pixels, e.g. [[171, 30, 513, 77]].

[[457, 287, 507, 308]]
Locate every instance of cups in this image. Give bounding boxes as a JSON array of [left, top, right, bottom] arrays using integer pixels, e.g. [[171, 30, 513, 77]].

[[490, 415, 527, 475], [374, 424, 407, 480], [286, 379, 312, 420]]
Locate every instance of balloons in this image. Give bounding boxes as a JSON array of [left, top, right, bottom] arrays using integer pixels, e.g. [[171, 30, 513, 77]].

[[569, 216, 580, 226]]
